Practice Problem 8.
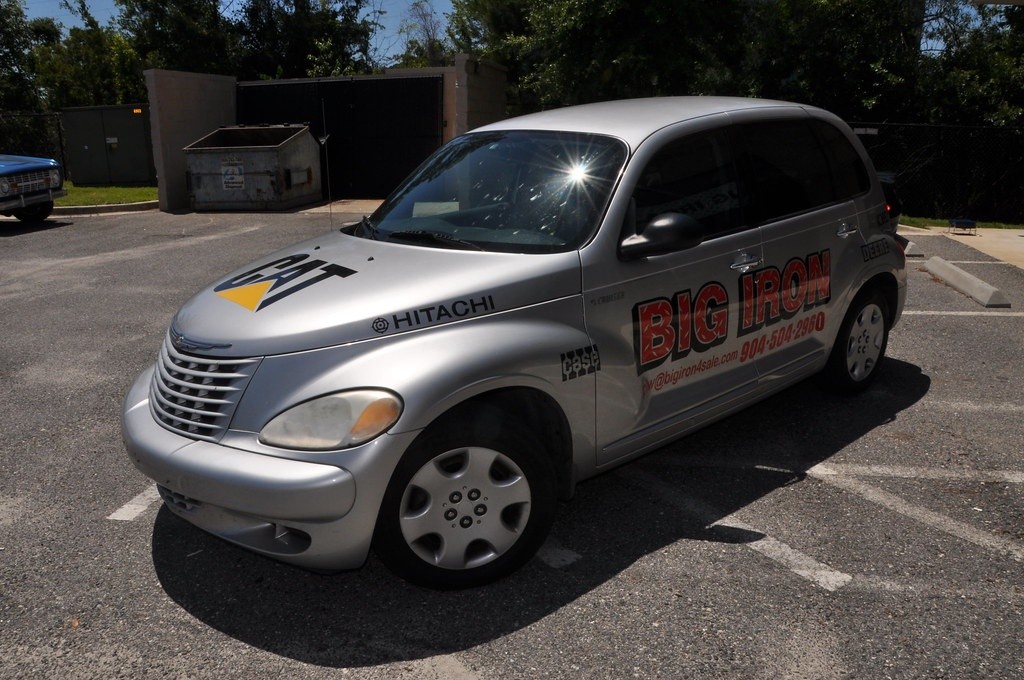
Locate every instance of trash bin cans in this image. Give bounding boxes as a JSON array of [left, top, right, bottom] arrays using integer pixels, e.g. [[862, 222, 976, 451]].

[[181, 123, 321, 208]]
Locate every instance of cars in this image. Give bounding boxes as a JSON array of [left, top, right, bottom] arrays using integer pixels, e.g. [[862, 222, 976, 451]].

[[0, 154, 68, 224], [121, 95, 911, 592]]
